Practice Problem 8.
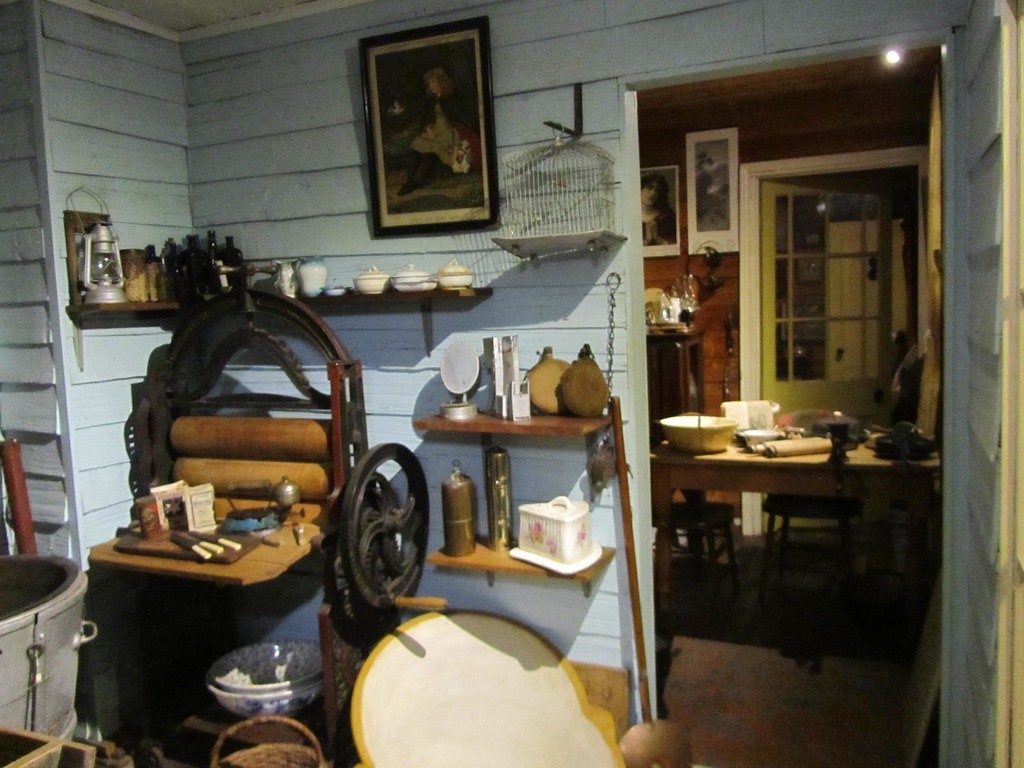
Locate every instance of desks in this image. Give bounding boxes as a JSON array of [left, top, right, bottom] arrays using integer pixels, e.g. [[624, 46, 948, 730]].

[[650, 439, 940, 648]]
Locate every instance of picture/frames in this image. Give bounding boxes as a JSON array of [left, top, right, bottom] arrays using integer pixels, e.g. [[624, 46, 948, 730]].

[[359, 14, 501, 239]]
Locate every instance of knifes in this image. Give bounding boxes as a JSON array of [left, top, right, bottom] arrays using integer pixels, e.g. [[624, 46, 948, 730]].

[[169, 532, 243, 561]]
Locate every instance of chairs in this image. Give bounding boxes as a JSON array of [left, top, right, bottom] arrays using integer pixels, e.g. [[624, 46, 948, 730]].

[[653, 412, 738, 601], [760, 408, 859, 600]]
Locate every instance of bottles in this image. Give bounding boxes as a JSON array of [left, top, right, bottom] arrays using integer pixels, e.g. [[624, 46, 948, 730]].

[[146, 230, 244, 301], [442, 458, 476, 558], [484, 446, 514, 552], [273, 260, 300, 300], [297, 255, 326, 297]]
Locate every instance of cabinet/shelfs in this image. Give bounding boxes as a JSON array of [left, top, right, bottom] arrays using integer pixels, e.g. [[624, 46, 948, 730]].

[[646, 325, 705, 454], [413, 395, 651, 726], [87, 292, 368, 768]]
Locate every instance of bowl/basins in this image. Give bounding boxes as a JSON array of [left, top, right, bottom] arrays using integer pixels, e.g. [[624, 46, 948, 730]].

[[351, 265, 475, 295], [659, 416, 738, 454], [205, 638, 325, 717]]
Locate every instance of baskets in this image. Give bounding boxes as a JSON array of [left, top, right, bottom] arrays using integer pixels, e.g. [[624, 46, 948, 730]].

[[209, 716, 328, 768]]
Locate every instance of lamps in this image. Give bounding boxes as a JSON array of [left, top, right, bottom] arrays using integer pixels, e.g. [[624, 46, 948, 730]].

[[686, 240, 724, 291]]
